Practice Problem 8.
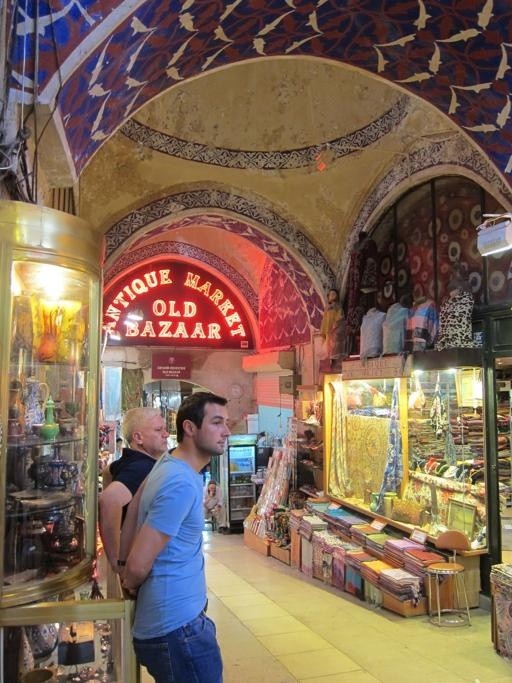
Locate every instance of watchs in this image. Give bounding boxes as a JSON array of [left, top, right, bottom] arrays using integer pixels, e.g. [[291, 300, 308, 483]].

[[116, 558, 126, 565]]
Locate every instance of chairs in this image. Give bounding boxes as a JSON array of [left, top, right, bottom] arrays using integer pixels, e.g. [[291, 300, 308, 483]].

[[427, 531, 474, 628]]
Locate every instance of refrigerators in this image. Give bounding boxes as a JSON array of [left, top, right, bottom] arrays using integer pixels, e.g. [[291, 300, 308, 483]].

[[223, 433, 257, 535]]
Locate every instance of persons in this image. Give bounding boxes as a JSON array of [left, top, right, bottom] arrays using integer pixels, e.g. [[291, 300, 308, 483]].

[[202, 480, 229, 534], [256, 432, 270, 447], [119, 392, 231, 683], [98, 407, 170, 593]]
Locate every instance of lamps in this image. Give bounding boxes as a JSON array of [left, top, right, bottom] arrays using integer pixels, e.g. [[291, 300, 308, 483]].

[[478, 212, 512, 258], [316, 142, 410, 182]]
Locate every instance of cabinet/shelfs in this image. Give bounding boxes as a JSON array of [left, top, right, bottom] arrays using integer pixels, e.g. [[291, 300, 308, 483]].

[[2, 201, 141, 683], [317, 347, 512, 618]]
